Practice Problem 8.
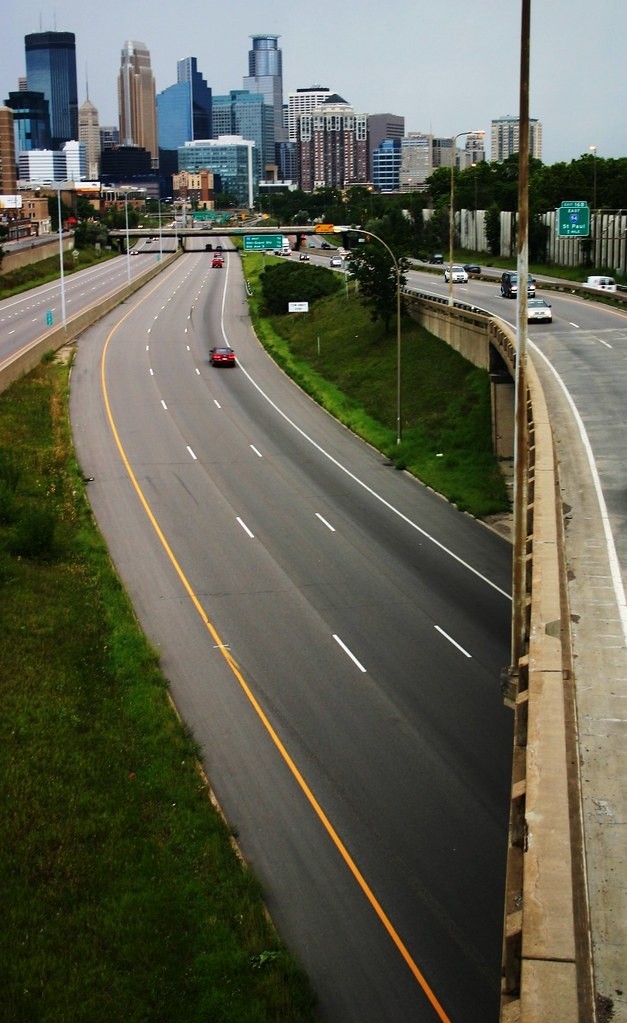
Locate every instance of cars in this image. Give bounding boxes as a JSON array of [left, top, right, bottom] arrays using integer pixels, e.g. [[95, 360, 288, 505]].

[[444, 265, 469, 284], [130, 249, 138, 254], [321, 242, 330, 249], [308, 243, 316, 248], [330, 255, 343, 267], [146, 237, 159, 244], [300, 253, 310, 261], [208, 345, 236, 368], [526, 299, 553, 324], [464, 264, 481, 275], [205, 243, 224, 268], [429, 253, 444, 264]]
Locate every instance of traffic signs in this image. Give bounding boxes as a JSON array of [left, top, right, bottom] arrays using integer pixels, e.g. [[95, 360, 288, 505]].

[[243, 235, 283, 251], [556, 200, 590, 237]]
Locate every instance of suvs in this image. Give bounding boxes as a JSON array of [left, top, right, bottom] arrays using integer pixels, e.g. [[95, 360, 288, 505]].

[[284, 245, 292, 257], [500, 271, 536, 299]]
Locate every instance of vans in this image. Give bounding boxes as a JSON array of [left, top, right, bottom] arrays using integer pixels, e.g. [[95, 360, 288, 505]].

[[586, 275, 617, 293]]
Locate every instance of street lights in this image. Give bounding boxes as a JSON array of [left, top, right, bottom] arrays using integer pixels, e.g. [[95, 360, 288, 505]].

[[30, 176, 87, 325], [447, 129, 487, 312], [332, 227, 402, 445], [266, 202, 270, 218], [106, 189, 148, 280], [254, 200, 262, 221], [589, 146, 597, 208], [145, 197, 173, 260]]
